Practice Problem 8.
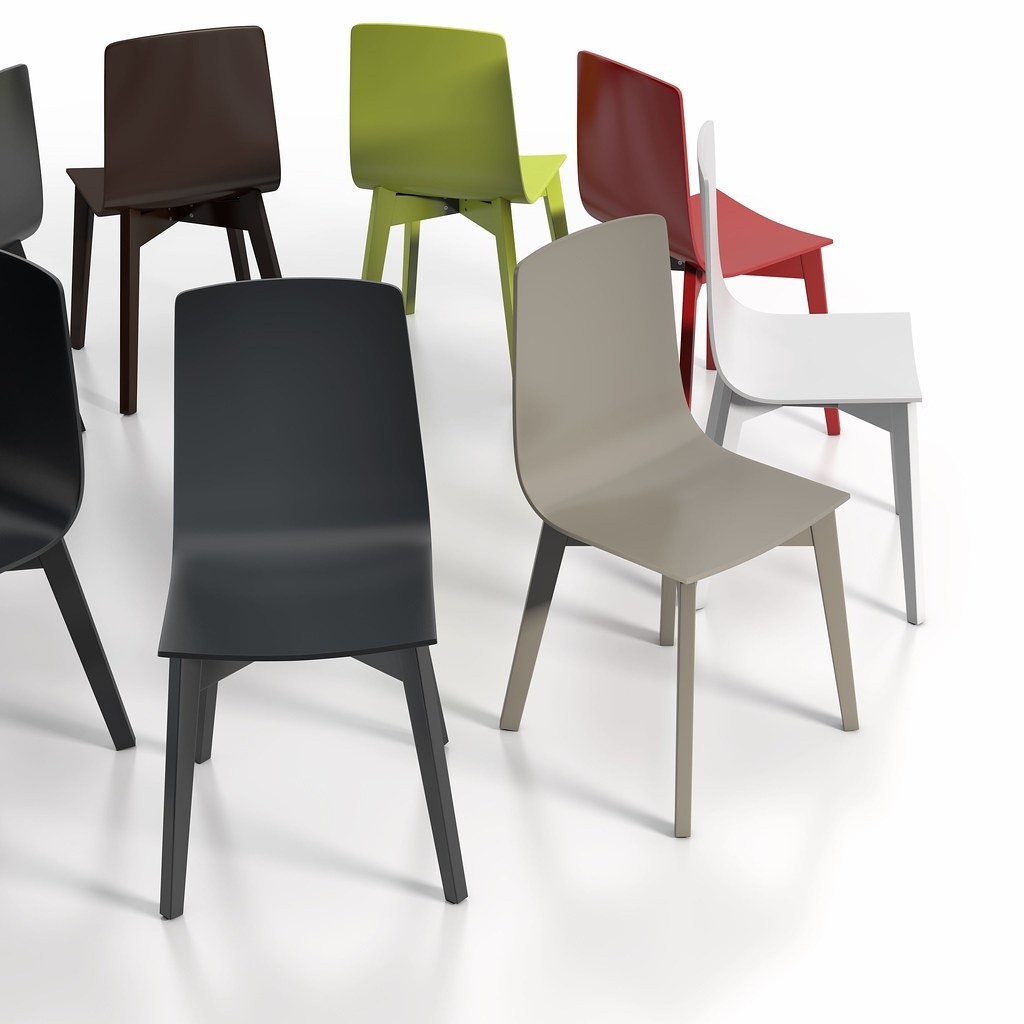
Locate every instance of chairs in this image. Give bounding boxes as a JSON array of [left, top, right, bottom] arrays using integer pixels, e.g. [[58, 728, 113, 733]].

[[156, 277, 467, 921], [0, 63, 44, 248], [569, 45, 843, 439], [348, 22, 567, 371], [0, 246, 141, 752], [65, 25, 284, 416], [695, 120, 922, 628], [499, 214, 860, 835]]
